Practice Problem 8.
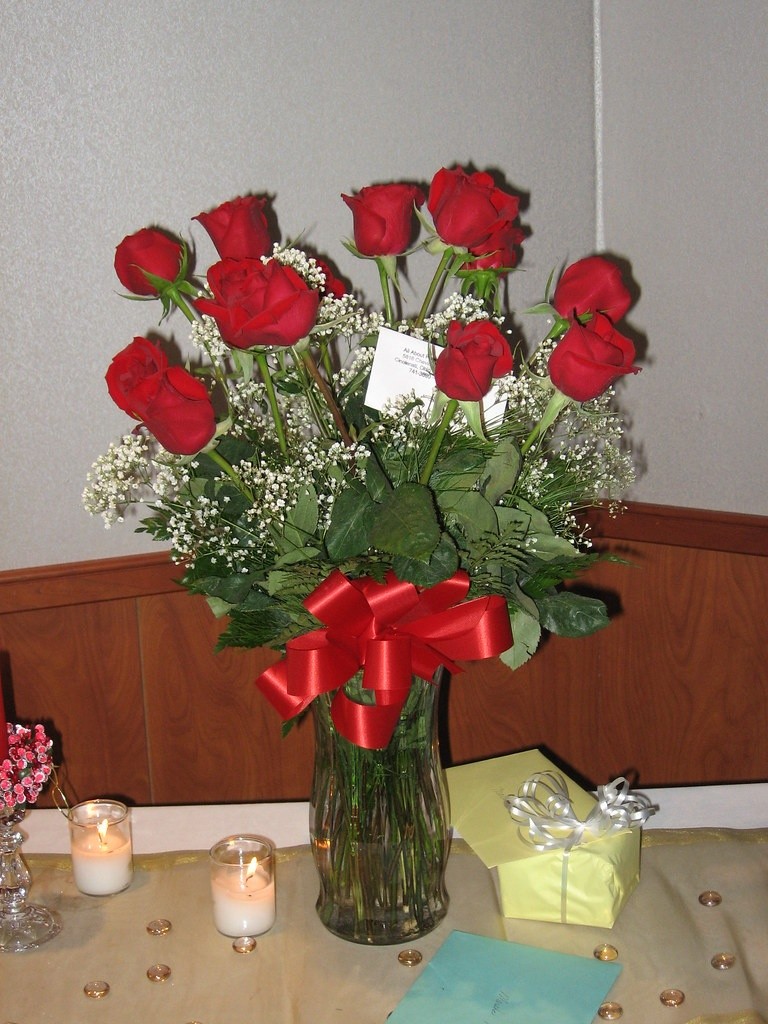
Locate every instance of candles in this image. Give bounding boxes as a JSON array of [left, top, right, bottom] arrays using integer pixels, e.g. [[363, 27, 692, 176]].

[[210, 836, 274, 939], [67, 799, 132, 897]]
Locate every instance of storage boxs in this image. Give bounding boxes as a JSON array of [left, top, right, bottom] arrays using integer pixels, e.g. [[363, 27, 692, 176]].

[[499, 823, 658, 927]]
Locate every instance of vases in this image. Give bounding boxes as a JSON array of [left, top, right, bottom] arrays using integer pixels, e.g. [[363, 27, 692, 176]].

[[308, 671, 452, 946]]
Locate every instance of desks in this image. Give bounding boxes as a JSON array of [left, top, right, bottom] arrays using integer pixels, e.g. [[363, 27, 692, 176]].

[[0, 780, 768, 1024]]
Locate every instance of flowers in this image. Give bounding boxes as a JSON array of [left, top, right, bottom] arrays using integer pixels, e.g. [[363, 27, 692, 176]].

[[0, 723, 55, 814], [81, 168, 642, 669]]
